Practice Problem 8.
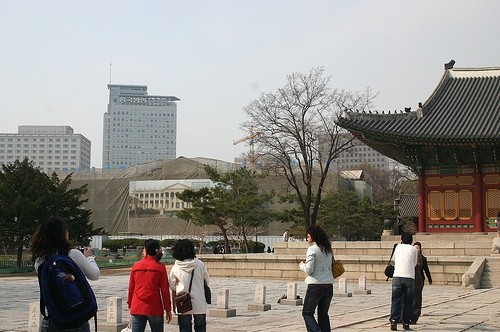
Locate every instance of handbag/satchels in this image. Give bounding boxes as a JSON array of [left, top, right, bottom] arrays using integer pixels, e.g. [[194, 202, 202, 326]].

[[332, 260, 345, 279], [121, 315, 132, 332], [175, 293, 192, 313], [385, 265, 394, 281]]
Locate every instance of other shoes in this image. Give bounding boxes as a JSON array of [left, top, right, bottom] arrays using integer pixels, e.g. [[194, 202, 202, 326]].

[[410, 320, 416, 324], [403, 323, 409, 329], [391, 323, 397, 330]]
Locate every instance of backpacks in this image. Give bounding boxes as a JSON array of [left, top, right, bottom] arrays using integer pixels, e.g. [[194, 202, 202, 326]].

[[37, 249, 98, 329]]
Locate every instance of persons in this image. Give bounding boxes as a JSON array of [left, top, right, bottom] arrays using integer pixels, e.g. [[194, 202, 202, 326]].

[[299, 226, 333, 332], [27, 216, 100, 332], [213, 244, 225, 254], [389, 231, 418, 330], [398, 242, 432, 324], [127, 239, 172, 332], [168, 239, 210, 332], [267, 246, 274, 253], [283, 230, 293, 242]]
